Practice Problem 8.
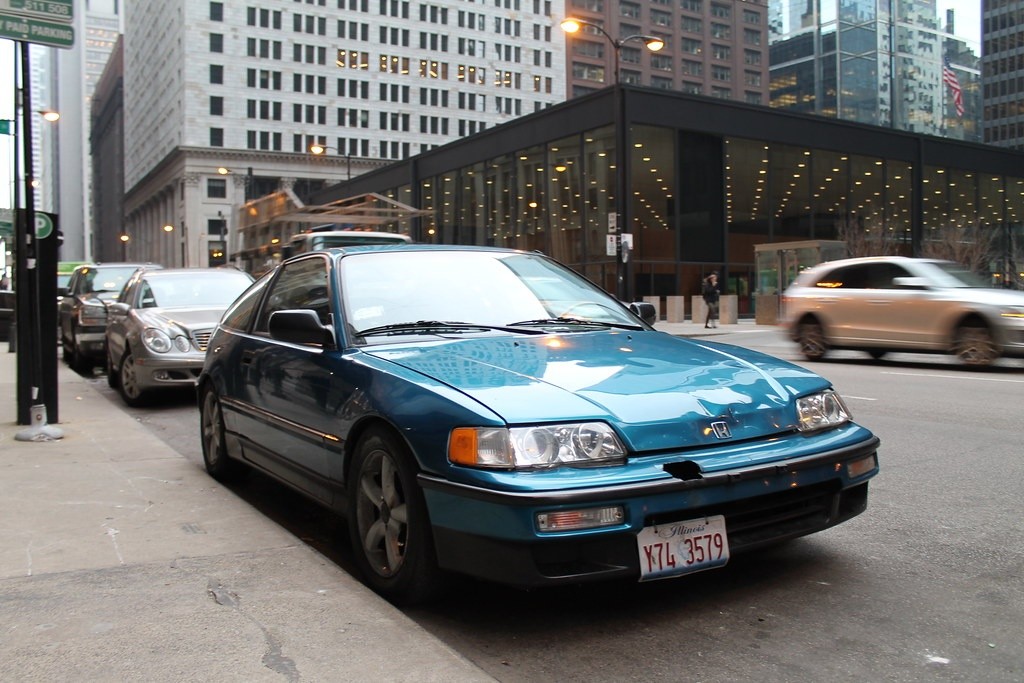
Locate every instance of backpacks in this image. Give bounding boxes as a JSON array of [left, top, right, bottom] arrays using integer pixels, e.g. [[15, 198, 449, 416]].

[[701, 279, 707, 296]]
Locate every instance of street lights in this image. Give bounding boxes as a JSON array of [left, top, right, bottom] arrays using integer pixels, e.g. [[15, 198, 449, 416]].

[[311, 144, 354, 230], [561, 18, 664, 300], [9, 107, 59, 353], [164, 224, 190, 268], [218, 167, 254, 201]]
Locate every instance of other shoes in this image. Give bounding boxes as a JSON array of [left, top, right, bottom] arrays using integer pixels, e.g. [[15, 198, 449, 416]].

[[704, 326, 710, 329], [712, 326, 716, 329]]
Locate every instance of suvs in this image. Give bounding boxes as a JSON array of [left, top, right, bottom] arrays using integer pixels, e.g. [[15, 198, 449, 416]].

[[280, 231, 412, 265], [57, 261, 99, 343], [779, 255, 1024, 368], [57, 264, 168, 373]]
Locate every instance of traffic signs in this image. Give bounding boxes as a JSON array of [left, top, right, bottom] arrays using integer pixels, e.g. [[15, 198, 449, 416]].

[[607, 213, 616, 233]]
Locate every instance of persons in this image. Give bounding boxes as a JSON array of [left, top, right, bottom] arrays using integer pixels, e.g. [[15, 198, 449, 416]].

[[702, 274, 718, 328]]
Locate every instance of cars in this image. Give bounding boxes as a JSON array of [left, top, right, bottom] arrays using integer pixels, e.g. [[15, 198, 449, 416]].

[[196, 244, 880, 610], [106, 265, 264, 408]]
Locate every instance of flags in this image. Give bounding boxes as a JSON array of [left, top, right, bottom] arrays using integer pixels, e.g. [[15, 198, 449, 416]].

[[944, 57, 964, 116]]
